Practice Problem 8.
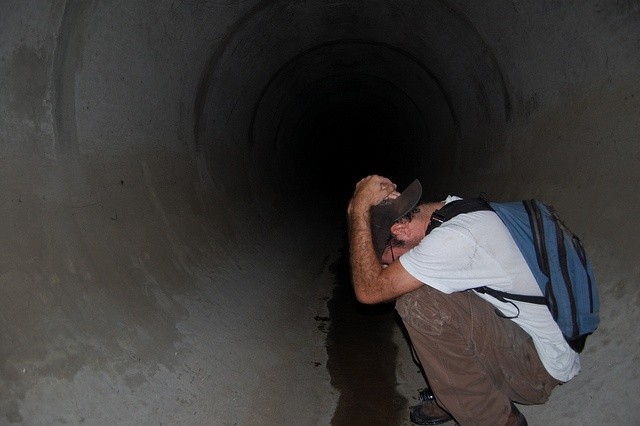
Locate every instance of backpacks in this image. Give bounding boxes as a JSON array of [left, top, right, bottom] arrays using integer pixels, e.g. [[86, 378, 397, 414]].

[[424, 196, 600, 353]]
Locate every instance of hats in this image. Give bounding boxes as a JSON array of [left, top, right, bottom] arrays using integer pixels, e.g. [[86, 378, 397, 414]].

[[369, 179, 422, 263]]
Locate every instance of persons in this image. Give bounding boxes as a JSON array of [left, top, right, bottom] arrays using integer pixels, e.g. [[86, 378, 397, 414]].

[[347, 175, 581, 426]]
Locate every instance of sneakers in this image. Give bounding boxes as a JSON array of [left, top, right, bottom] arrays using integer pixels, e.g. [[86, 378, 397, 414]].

[[410, 399, 453, 425], [506, 403, 529, 426]]
